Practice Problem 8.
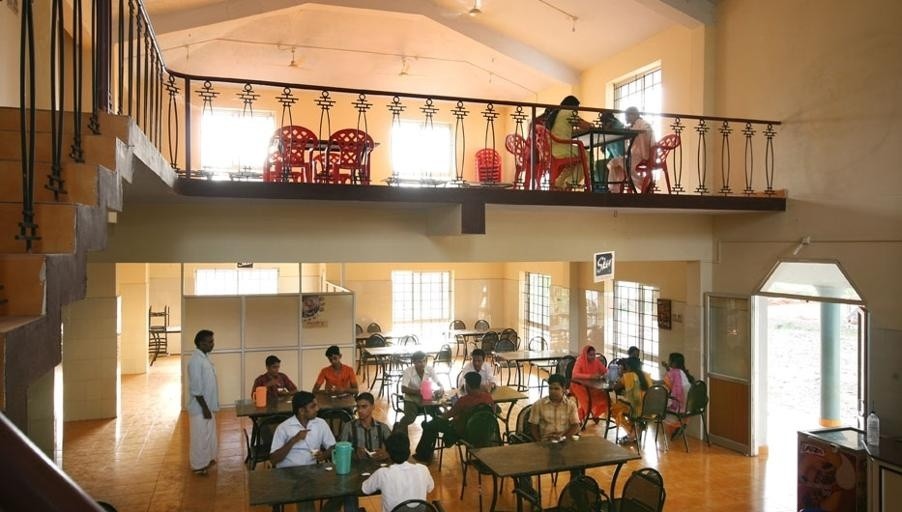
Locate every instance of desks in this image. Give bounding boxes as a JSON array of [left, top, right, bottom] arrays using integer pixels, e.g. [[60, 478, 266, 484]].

[[468, 435, 641, 510], [248, 460, 389, 511], [235, 389, 357, 470], [797, 426, 902, 511], [568, 377, 666, 441]]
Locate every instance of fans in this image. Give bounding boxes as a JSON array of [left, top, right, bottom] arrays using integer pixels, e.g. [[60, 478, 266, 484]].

[[267, 47, 313, 72], [444, 0, 493, 18], [379, 57, 428, 80]]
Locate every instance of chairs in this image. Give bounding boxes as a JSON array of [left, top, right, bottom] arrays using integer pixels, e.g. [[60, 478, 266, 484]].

[[517, 405, 562, 488], [473, 123, 681, 196], [393, 500, 448, 511], [566, 360, 605, 427], [320, 129, 373, 187], [318, 409, 353, 453], [615, 385, 670, 453], [264, 126, 316, 184], [353, 321, 578, 470], [513, 476, 602, 511], [655, 379, 708, 453], [602, 468, 666, 512], [257, 416, 322, 511], [610, 358, 623, 373], [460, 411, 518, 509]]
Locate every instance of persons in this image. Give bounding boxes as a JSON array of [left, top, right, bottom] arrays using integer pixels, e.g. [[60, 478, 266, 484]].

[[529, 95, 656, 194], [460, 348, 496, 392], [399, 351, 445, 424], [361, 431, 434, 512], [339, 392, 392, 512], [268, 390, 343, 511], [311, 345, 360, 397], [411, 371, 496, 461], [569, 344, 695, 444], [518, 374, 585, 496], [186, 329, 219, 476], [250, 354, 299, 444]]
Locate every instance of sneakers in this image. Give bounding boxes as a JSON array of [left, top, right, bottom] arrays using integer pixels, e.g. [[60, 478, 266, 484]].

[[591, 411, 599, 425], [412, 453, 433, 460], [619, 435, 636, 445], [670, 424, 688, 441]]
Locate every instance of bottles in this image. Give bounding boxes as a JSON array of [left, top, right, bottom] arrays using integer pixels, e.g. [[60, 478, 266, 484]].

[[331, 440, 355, 475], [253, 386, 267, 407], [605, 360, 619, 382], [866, 410, 880, 447], [420, 378, 433, 400]]
